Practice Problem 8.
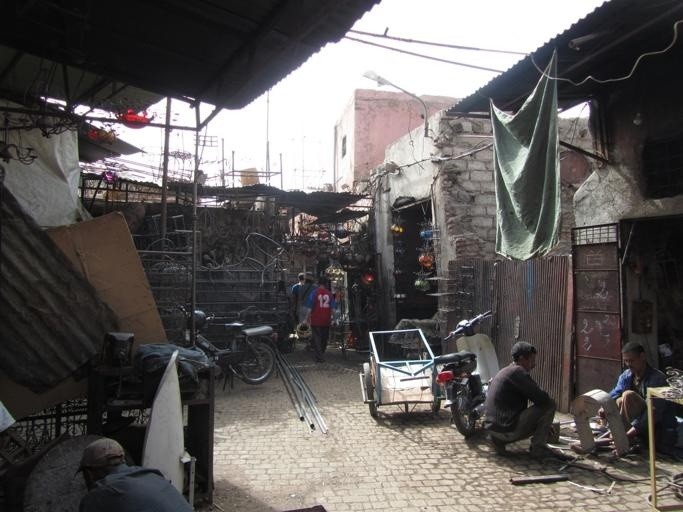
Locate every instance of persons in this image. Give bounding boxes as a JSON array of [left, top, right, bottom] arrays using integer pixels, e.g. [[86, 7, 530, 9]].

[[291, 273, 311, 329], [75, 438, 194, 512], [598, 341, 678, 455], [307, 276, 337, 362], [482, 341, 557, 456]]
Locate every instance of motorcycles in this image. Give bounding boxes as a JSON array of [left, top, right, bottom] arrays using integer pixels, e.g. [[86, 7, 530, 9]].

[[434, 309, 500, 438], [175, 302, 276, 391]]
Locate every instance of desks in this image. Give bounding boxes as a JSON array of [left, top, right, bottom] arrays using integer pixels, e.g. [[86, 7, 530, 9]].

[[646, 385, 683, 509]]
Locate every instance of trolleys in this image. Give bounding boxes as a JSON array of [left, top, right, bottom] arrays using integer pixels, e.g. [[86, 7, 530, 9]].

[[358, 328, 441, 418]]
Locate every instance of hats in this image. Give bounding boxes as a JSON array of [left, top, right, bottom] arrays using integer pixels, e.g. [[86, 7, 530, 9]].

[[306, 272, 314, 281], [78, 436, 128, 470]]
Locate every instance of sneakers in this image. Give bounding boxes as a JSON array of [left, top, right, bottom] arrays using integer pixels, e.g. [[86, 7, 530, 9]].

[[485, 434, 506, 454], [531, 445, 563, 456], [315, 346, 327, 360]]
